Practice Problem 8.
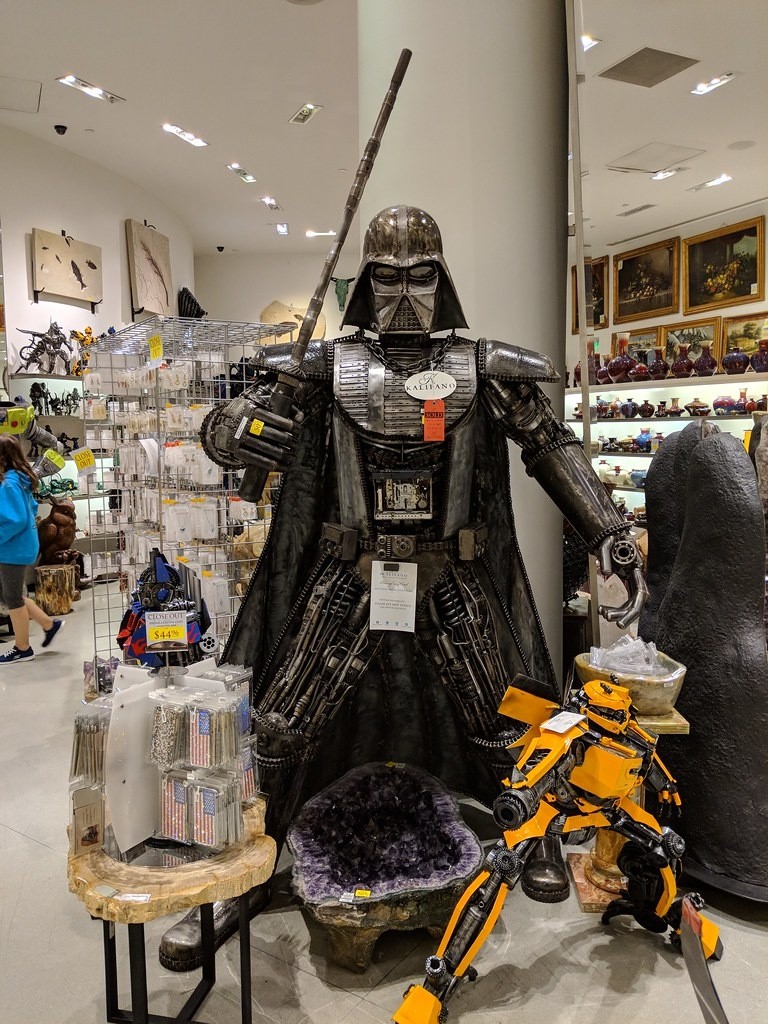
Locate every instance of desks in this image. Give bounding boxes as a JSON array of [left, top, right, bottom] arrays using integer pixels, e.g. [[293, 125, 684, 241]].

[[65, 798, 277, 1024]]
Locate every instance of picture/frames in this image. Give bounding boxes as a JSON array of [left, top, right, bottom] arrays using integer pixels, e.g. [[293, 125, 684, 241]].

[[659, 316, 720, 379], [682, 216, 765, 316], [571, 255, 609, 335], [722, 311, 768, 372], [611, 326, 661, 365], [613, 236, 680, 325]]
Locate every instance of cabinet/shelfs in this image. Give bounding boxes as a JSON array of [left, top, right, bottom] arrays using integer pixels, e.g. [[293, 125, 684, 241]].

[[564, 372, 768, 493], [9, 372, 118, 554]]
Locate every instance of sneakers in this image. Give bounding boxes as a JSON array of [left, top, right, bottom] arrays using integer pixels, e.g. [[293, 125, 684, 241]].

[[42, 619, 65, 647], [0, 646, 35, 664]]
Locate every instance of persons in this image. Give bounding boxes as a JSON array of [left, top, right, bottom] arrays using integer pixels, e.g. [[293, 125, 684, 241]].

[[158, 206, 650, 972], [0, 435, 65, 665]]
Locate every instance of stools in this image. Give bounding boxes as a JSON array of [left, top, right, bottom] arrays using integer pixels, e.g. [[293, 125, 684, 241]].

[[34, 564, 75, 616]]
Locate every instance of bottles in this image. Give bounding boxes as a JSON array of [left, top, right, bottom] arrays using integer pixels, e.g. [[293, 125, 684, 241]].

[[572, 332, 768, 523]]
